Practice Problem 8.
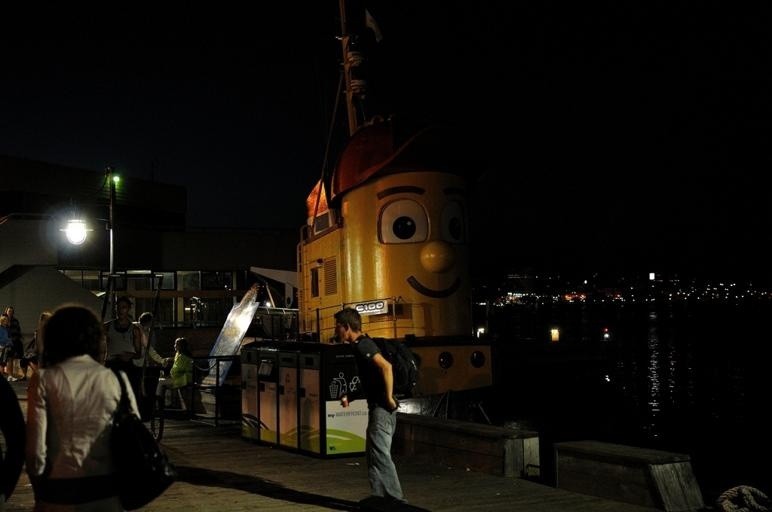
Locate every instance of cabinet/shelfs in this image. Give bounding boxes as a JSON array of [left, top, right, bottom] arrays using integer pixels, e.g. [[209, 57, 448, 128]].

[[190, 353, 241, 433]]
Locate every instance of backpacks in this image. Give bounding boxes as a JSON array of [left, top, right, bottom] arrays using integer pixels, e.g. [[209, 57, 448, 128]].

[[370, 337, 418, 395]]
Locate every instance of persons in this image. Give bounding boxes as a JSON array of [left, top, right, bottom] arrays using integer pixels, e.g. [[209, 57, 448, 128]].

[[334, 307, 409, 511], [1, 294, 195, 510]]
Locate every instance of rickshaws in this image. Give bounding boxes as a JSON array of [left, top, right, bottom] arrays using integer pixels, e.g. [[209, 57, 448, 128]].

[[98, 272, 168, 444]]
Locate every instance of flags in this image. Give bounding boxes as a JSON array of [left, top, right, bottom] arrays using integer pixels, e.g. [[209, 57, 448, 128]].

[[363, 10, 383, 42]]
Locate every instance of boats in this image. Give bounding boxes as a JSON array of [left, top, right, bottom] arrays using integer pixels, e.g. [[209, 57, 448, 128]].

[[293, 2, 495, 422]]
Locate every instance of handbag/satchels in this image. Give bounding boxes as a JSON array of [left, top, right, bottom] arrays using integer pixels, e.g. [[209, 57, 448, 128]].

[[108, 408, 177, 511]]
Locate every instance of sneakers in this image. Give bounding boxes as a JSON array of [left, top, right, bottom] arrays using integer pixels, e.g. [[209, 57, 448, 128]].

[[8, 376, 18, 382], [360, 495, 403, 506]]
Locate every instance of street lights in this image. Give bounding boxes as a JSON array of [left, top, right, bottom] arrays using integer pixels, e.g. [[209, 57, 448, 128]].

[[55, 164, 120, 334]]
